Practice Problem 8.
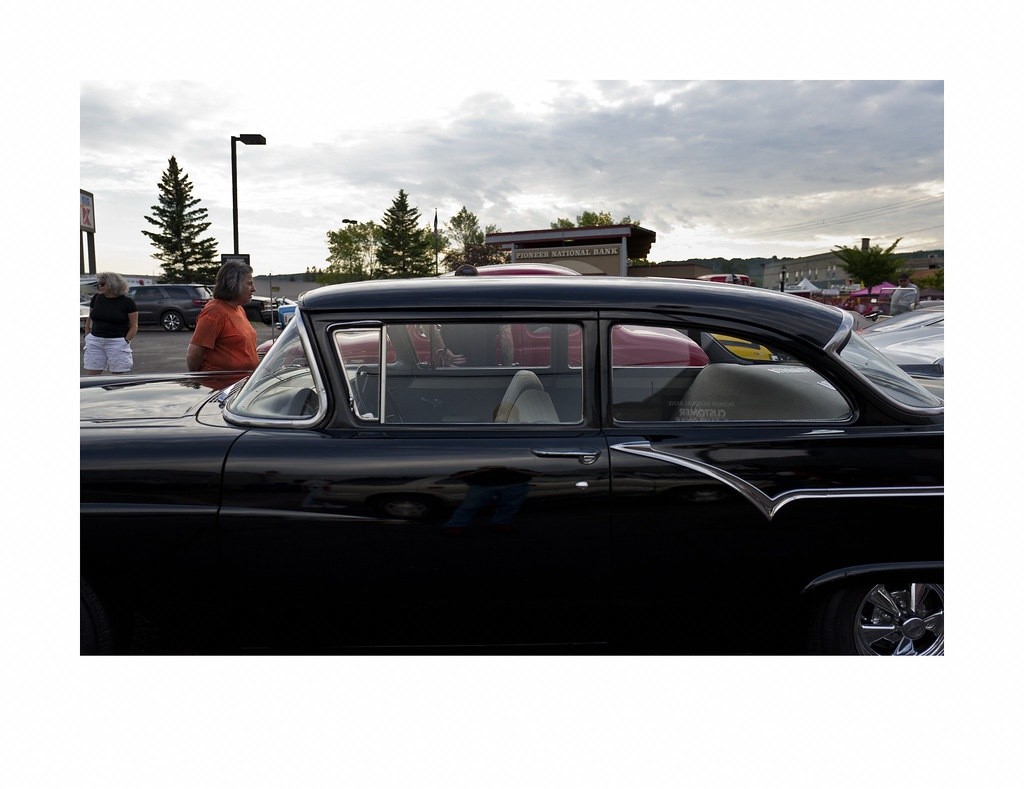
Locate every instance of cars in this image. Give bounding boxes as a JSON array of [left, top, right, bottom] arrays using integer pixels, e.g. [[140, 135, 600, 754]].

[[253, 260, 709, 370], [242, 292, 296, 322], [857, 298, 944, 404], [81, 272, 943, 656]]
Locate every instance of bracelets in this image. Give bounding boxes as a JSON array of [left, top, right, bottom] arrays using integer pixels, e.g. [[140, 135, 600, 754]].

[[125, 337, 129, 343]]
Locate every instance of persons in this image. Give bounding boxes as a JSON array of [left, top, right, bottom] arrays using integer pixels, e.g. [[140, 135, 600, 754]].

[[186, 260, 260, 372], [890, 273, 920, 316], [421, 264, 515, 367], [84, 272, 138, 375], [833, 299, 858, 311]]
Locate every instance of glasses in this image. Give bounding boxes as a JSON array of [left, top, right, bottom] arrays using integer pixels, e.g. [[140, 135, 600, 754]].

[[98, 281, 107, 287]]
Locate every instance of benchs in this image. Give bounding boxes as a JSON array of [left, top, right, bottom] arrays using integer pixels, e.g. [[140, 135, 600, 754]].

[[673, 362, 850, 423]]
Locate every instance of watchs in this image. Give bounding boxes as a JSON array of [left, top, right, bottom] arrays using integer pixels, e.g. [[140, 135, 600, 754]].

[[436, 347, 448, 355]]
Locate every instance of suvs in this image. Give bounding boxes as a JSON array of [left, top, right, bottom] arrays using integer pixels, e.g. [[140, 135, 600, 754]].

[[128, 284, 213, 331]]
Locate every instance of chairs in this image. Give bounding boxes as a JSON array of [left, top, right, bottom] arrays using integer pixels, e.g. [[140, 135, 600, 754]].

[[495, 370, 563, 423], [140, 290, 160, 297]]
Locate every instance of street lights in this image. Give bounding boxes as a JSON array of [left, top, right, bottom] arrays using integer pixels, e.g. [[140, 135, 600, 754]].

[[777, 263, 788, 291], [231, 134, 267, 254]]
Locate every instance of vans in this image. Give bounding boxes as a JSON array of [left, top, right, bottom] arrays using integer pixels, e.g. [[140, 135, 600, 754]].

[[705, 273, 751, 285]]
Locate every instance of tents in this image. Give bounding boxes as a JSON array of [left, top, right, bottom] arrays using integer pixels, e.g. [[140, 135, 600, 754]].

[[848, 280, 898, 297], [779, 278, 822, 293]]
